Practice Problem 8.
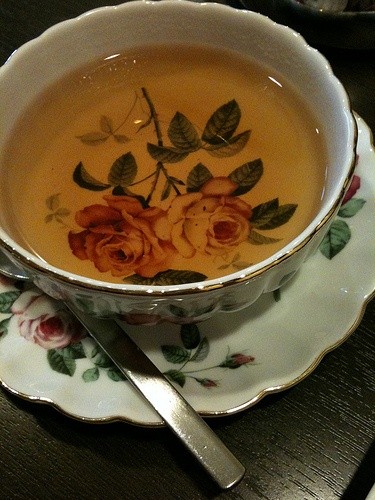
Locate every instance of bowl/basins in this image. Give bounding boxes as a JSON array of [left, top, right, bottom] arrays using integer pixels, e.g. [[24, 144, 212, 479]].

[[0, 0, 358, 327]]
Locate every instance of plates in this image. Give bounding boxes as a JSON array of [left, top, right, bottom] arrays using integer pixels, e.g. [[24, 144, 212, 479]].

[[1, 108, 375, 427]]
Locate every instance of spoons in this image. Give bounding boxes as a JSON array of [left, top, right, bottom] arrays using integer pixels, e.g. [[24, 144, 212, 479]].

[[0, 245, 247, 492]]
